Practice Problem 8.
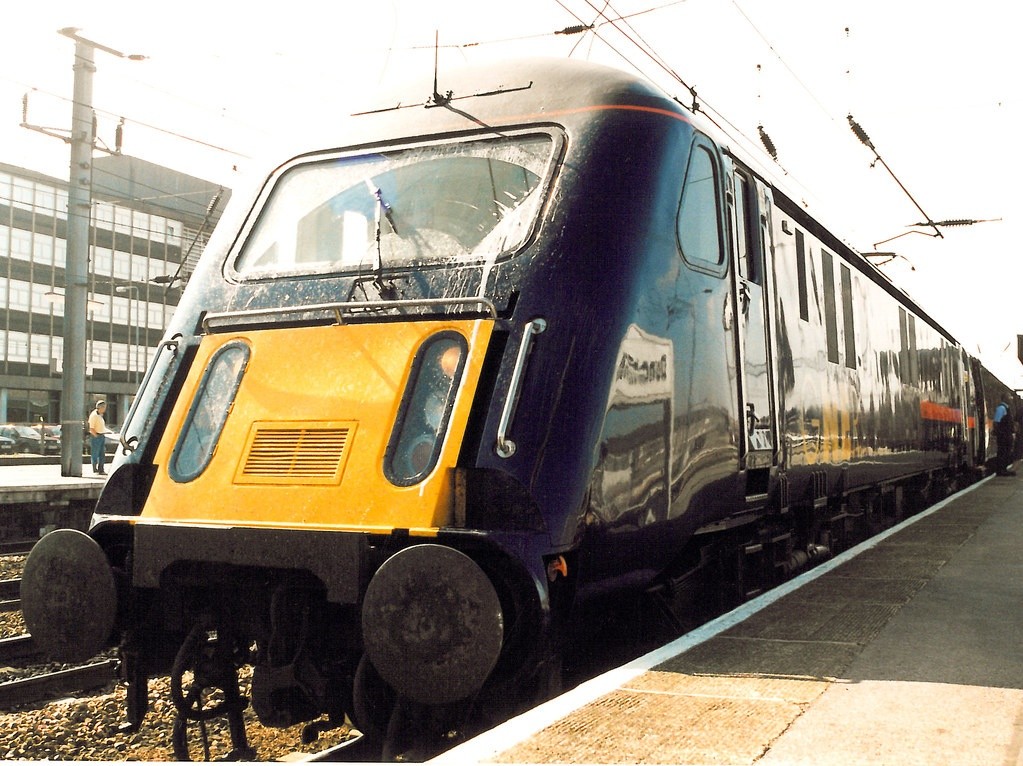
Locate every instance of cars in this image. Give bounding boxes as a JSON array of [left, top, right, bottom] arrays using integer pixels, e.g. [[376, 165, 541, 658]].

[[0, 423, 122, 455]]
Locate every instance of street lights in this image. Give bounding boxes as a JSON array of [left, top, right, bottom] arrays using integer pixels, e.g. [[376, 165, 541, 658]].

[[115, 285, 149, 395]]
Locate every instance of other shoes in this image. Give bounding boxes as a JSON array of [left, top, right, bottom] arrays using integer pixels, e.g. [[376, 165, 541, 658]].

[[98, 465, 108, 475], [93, 465, 98, 472], [996, 467, 1016, 477]]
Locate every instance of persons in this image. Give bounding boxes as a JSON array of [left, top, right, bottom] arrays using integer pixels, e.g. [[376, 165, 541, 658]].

[[991, 392, 1016, 477], [88, 399, 109, 476]]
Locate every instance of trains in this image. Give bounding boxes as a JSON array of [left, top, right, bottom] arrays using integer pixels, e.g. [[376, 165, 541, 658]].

[[16, 53, 1023, 763]]
